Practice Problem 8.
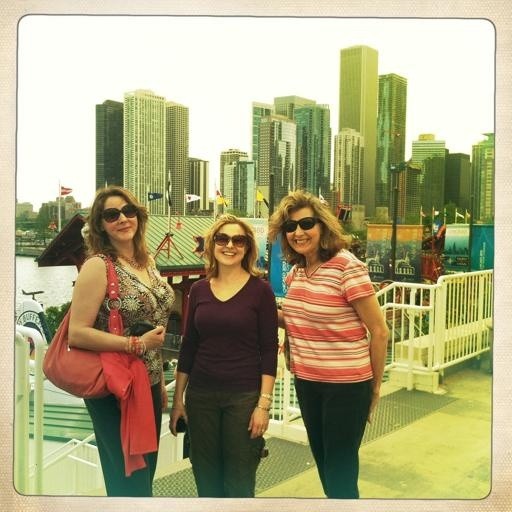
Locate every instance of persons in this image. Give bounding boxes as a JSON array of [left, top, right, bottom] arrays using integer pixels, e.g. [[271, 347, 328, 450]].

[[69, 186, 177, 499], [168, 213, 280, 497], [267, 191, 390, 499]]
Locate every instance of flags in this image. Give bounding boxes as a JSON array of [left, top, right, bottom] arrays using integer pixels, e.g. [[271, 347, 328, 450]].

[[61, 187, 72, 196], [186, 194, 202, 203], [148, 192, 164, 201], [256, 190, 270, 208], [217, 189, 230, 208], [320, 195, 328, 203], [420, 211, 471, 219], [166, 179, 173, 206]]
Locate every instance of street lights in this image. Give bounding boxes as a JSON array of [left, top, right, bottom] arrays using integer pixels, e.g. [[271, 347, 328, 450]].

[[388, 162, 399, 282]]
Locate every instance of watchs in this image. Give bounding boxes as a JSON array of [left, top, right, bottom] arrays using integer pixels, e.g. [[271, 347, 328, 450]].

[[260, 392, 276, 400]]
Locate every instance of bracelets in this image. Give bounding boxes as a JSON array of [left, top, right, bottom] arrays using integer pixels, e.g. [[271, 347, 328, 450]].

[[255, 403, 272, 411], [126, 335, 146, 357]]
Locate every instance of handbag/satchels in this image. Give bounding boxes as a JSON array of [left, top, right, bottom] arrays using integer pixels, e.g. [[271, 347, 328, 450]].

[[43, 309, 126, 400], [285, 328, 289, 369]]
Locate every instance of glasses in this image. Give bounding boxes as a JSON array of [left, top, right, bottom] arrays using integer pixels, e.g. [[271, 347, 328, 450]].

[[101, 203, 137, 223], [281, 217, 322, 232], [212, 233, 248, 248]]
